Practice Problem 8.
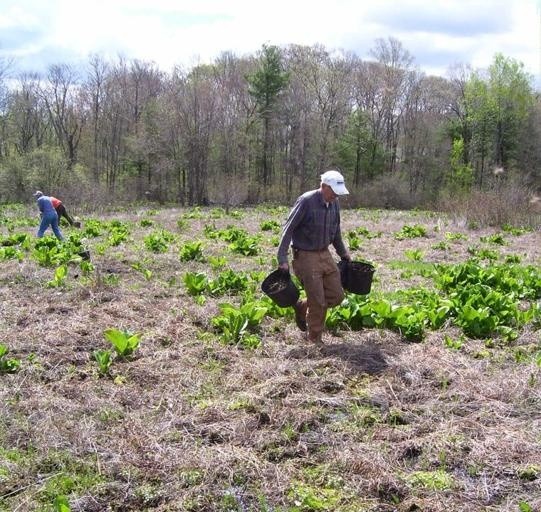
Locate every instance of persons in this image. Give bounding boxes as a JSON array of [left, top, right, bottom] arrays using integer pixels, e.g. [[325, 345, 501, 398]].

[[277, 170, 353, 347], [49, 195, 83, 228], [33, 191, 67, 242]]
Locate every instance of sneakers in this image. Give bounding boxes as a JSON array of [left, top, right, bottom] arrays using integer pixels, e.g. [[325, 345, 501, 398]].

[[292, 300, 307, 331]]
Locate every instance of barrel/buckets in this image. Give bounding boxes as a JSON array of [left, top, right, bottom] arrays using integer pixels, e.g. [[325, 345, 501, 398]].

[[337, 260, 377, 294], [261, 270, 300, 308]]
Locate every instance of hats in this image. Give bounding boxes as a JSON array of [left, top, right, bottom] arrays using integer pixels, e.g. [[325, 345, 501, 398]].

[[32, 191, 43, 197], [321, 170, 350, 196]]
